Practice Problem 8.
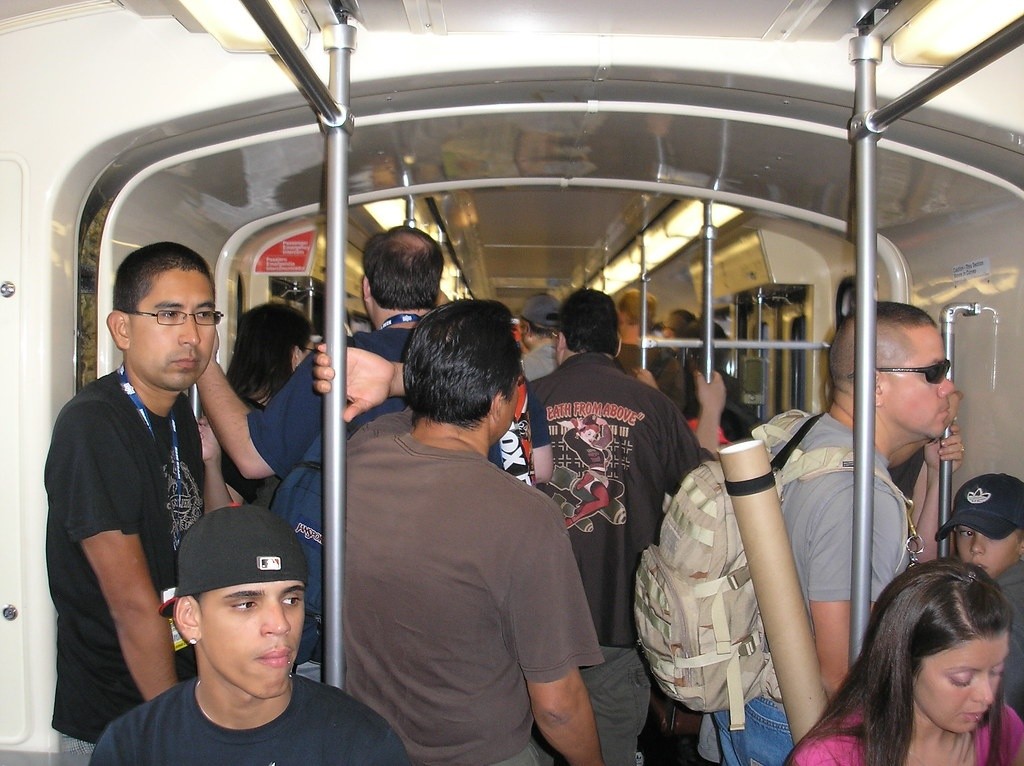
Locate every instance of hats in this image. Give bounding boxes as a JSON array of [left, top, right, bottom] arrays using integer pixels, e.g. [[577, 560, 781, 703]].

[[932, 472, 1024, 542], [159, 504, 309, 619], [512, 293, 562, 329]]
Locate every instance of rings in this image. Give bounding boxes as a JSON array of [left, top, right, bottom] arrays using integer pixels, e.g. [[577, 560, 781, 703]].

[[960, 444, 966, 452]]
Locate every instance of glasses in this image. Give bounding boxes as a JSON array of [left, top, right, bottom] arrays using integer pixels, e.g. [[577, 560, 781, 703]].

[[848, 358, 951, 384], [117, 307, 224, 325]]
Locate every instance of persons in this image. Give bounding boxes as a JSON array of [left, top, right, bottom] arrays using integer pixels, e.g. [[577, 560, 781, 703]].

[[785, 558, 1024, 766], [43, 242, 227, 745], [195, 226, 501, 480], [511, 292, 562, 383], [715, 300, 953, 766], [92, 502, 411, 766], [198, 301, 315, 513], [315, 298, 605, 766], [613, 289, 759, 443], [523, 289, 726, 766], [888, 425, 1024, 718]]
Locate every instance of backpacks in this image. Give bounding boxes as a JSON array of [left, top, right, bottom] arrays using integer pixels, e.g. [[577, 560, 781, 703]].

[[632, 408, 910, 730]]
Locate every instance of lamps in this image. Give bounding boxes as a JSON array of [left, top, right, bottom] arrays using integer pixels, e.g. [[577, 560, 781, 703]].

[[891, 0, 1024, 69], [177, 0, 312, 56], [587, 197, 743, 298]]
